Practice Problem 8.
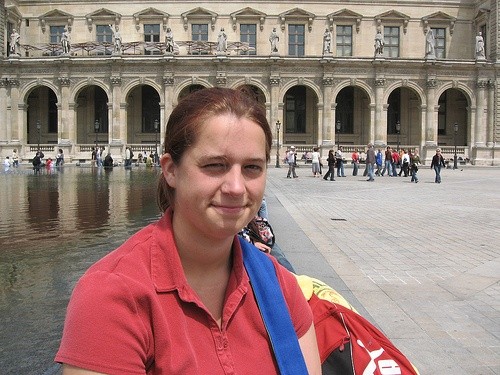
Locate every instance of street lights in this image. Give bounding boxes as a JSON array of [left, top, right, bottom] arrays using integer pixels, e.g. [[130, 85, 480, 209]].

[[276, 120, 280, 167], [335, 119, 340, 149], [154, 119, 158, 153], [37, 119, 40, 152], [95, 119, 99, 146], [452, 121, 458, 169], [395, 119, 400, 152]]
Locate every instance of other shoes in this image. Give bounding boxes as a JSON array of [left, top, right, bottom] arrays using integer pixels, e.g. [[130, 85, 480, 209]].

[[330, 179, 335, 181], [367, 178, 374, 181], [287, 176, 291, 178], [293, 175, 298, 178], [323, 176, 327, 180], [397, 174, 401, 176], [415, 180, 419, 183]]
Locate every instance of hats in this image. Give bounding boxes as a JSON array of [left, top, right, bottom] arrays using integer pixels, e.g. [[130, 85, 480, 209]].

[[290, 146, 295, 148]]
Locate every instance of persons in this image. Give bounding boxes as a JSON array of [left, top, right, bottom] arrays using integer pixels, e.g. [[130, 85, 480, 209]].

[[54, 149, 64, 166], [352, 148, 360, 176], [218, 27, 227, 52], [336, 146, 346, 177], [363, 147, 417, 177], [9, 28, 20, 52], [11, 150, 19, 165], [90, 145, 115, 166], [113, 25, 122, 52], [60, 28, 70, 54], [60, 86, 322, 375], [375, 30, 384, 54], [366, 143, 376, 182], [32, 147, 54, 167], [425, 29, 435, 55], [310, 147, 324, 178], [5, 156, 11, 167], [324, 28, 333, 53], [286, 146, 299, 179], [323, 149, 337, 181], [410, 150, 421, 183], [123, 146, 158, 166], [430, 148, 445, 184], [269, 27, 279, 52], [474, 31, 484, 57], [165, 28, 174, 51]]
